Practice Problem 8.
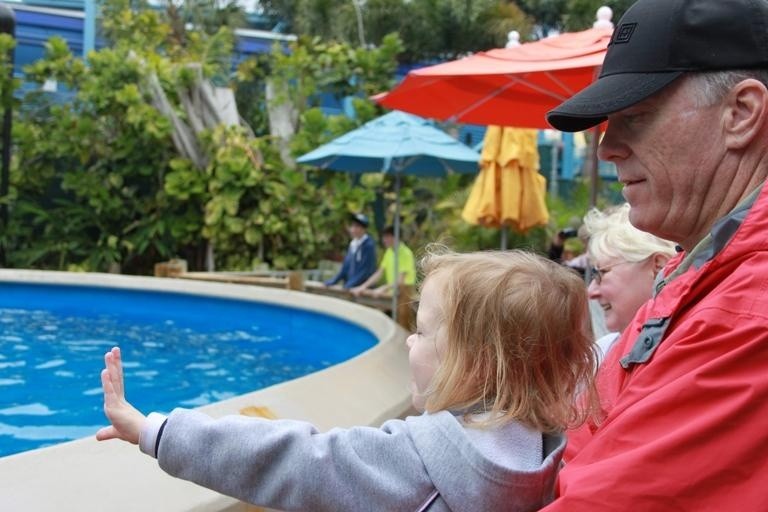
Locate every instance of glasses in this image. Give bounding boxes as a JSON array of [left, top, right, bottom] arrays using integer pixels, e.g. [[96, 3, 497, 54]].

[[591, 262, 622, 284]]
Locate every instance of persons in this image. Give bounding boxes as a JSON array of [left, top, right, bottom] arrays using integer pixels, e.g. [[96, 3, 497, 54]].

[[545, 203, 682, 394], [545, 0, 768, 511], [321, 212, 378, 290], [349, 225, 418, 299], [94, 240, 606, 510]]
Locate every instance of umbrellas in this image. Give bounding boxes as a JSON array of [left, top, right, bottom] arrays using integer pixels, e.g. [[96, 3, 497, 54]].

[[293, 107, 483, 320], [463, 29, 552, 252], [366, 1, 621, 211]]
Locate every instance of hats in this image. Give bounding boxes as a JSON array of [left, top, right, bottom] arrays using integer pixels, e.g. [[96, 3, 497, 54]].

[[348, 213, 369, 228], [546, 0, 766, 132]]
